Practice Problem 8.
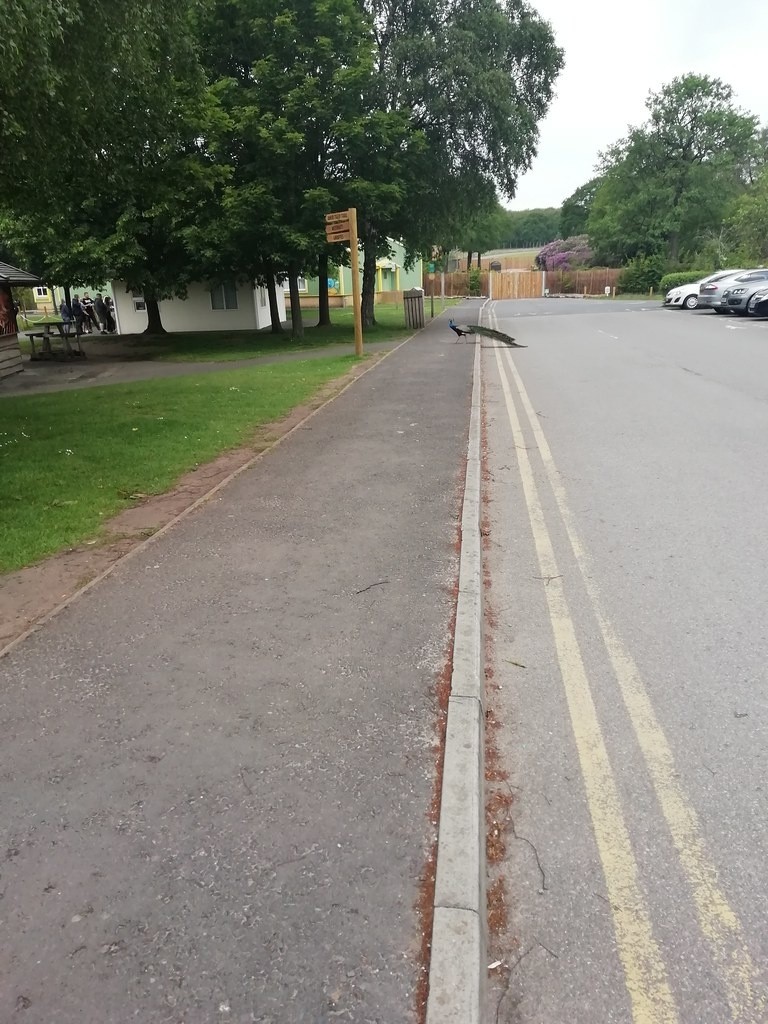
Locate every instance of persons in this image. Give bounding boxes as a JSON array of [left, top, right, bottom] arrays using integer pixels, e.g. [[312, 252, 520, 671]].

[[59, 292, 117, 335], [12, 298, 20, 333]]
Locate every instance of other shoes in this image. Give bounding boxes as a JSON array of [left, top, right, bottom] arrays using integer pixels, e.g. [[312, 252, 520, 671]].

[[101, 331, 107, 334]]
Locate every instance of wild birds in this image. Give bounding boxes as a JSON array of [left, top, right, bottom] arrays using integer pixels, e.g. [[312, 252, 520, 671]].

[[449, 318, 525, 348]]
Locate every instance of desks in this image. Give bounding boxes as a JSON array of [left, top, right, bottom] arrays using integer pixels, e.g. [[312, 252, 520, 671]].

[[33, 322, 77, 359]]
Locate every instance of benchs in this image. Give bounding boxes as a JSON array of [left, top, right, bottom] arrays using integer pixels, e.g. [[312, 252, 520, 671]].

[[25, 331, 85, 362]]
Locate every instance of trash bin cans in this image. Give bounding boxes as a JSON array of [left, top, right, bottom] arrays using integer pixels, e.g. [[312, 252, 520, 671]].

[[403, 287, 425, 328]]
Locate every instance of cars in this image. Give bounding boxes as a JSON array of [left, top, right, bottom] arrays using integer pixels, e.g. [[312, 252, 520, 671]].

[[665, 269, 746, 310], [697, 269, 768, 315], [721, 279, 768, 315], [747, 288, 768, 316]]
